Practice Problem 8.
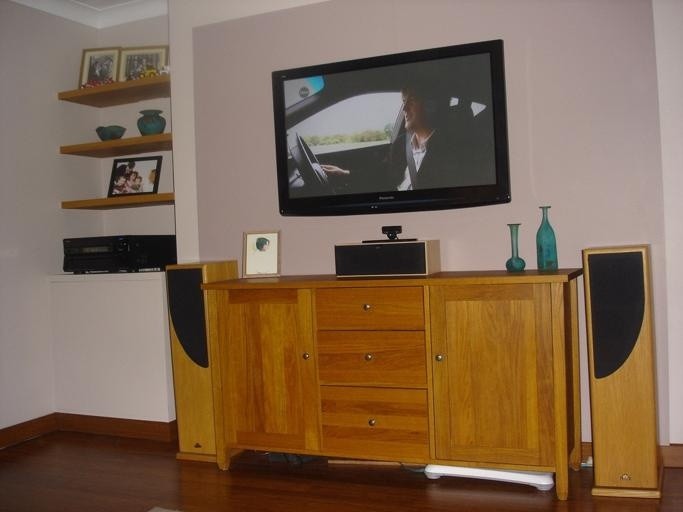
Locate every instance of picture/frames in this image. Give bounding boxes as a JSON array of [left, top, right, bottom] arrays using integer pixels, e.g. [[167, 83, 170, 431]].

[[242, 230, 281, 278], [106, 155, 162, 198], [117, 45, 169, 82], [79, 47, 120, 88]]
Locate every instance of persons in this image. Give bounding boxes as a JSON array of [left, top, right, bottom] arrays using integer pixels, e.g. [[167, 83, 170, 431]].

[[123, 171, 144, 193], [116, 160, 136, 176], [319, 77, 452, 194], [112, 175, 129, 194], [249, 237, 276, 273], [130, 176, 142, 193]]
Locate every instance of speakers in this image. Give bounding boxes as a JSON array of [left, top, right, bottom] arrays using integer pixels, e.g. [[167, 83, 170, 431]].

[[165, 260, 244, 461], [582, 245, 664, 499]]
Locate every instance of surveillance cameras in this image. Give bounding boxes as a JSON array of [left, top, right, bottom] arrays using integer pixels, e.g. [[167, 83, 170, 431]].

[[382, 225, 401, 243]]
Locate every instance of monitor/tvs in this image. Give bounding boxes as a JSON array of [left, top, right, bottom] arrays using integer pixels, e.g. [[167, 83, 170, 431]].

[[272, 39, 512, 216]]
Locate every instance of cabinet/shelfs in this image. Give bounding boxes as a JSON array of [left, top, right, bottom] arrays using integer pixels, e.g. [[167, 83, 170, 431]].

[[429, 283, 557, 470], [201, 269, 587, 502], [205, 288, 317, 451]]
